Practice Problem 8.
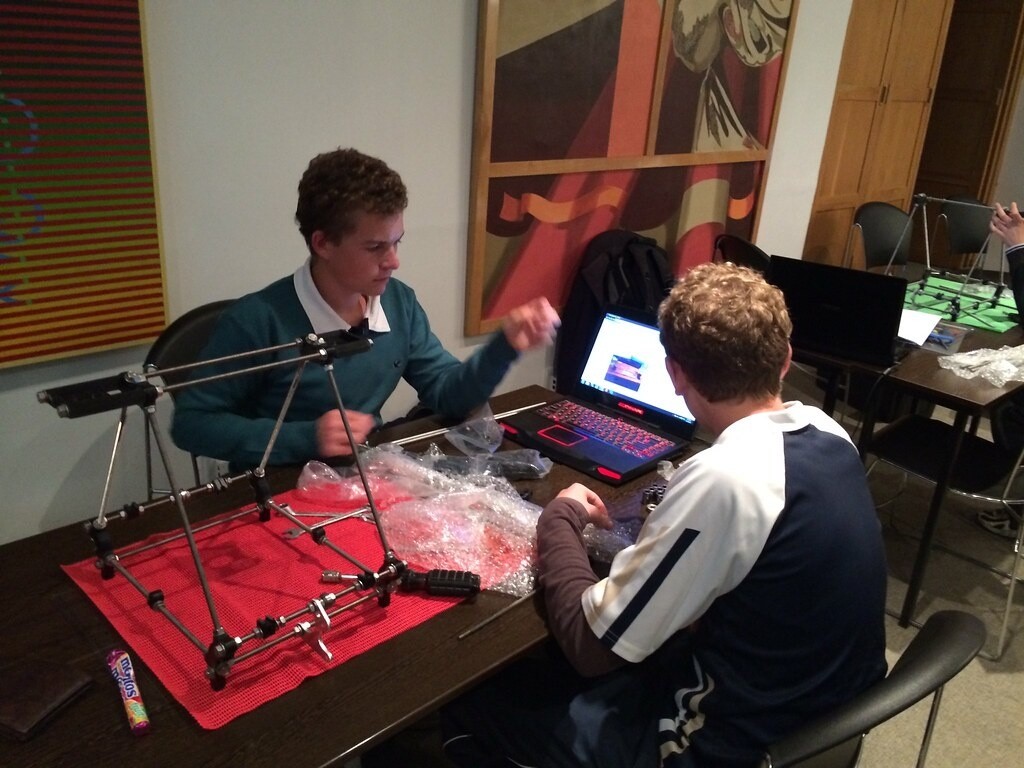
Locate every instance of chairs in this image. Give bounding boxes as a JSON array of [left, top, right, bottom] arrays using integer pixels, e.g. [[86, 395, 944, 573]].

[[556, 229, 697, 442], [930, 192, 994, 268], [764, 610, 987, 768], [839, 201, 913, 270], [142, 300, 236, 500], [711, 235, 771, 271], [856, 413, 1024, 662]]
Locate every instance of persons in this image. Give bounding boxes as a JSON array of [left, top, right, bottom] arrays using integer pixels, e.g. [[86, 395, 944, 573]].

[[990, 201, 1024, 325], [172, 148, 561, 475], [536, 261, 890, 768]]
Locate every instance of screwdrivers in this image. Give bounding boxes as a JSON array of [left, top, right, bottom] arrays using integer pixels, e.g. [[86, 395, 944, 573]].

[[320, 568, 480, 597]]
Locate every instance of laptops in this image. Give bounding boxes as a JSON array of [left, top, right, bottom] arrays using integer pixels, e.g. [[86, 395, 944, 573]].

[[767, 255, 915, 368], [494, 305, 698, 488]]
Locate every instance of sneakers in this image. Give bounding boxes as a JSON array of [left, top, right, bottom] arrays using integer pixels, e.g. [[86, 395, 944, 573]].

[[979, 505, 1024, 540]]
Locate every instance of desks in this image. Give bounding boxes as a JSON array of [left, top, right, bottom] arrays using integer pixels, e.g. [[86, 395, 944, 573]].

[[0, 384, 710, 768], [792, 261, 1024, 630]]
